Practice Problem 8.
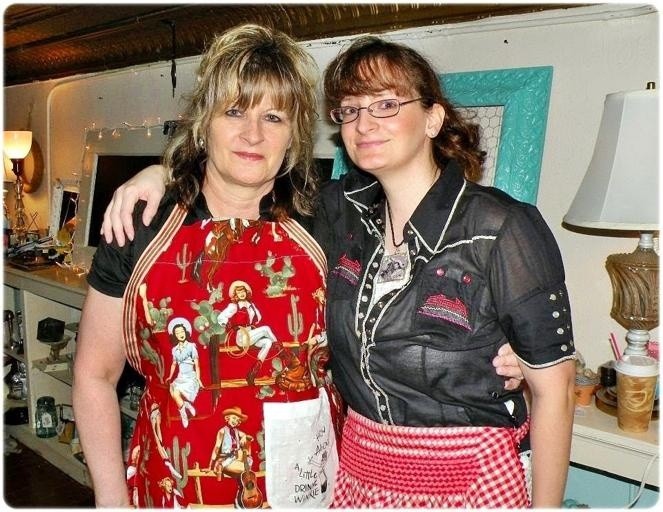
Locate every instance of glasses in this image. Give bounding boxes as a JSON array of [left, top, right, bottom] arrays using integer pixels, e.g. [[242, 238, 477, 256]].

[[329, 96, 422, 125]]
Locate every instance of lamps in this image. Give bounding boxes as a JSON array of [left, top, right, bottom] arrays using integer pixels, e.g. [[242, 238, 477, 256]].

[[561, 82, 660, 434], [2, 129, 32, 245]]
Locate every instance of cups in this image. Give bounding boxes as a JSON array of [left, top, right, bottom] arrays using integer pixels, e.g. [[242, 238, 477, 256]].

[[613, 354, 660, 434]]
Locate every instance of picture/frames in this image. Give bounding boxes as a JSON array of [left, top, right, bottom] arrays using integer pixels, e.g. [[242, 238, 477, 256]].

[[331, 65, 552, 207], [73, 122, 338, 271]]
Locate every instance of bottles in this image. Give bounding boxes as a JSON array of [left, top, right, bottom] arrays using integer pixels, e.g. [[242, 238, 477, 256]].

[[34, 396, 59, 439], [3, 309, 24, 356]]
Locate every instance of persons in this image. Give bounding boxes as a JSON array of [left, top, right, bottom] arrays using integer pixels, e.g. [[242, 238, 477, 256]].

[[71, 25, 342, 509], [99, 36, 577, 509]]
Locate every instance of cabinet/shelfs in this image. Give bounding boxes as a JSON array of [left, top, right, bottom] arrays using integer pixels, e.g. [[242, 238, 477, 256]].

[[3, 268, 144, 491]]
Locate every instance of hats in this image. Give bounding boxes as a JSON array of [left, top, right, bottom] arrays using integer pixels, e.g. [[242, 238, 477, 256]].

[[168, 318, 192, 336], [222, 407, 247, 420], [228, 281, 252, 298]]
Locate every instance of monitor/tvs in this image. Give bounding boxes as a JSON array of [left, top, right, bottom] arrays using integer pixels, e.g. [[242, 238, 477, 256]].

[[258, 119, 343, 232], [71, 129, 175, 268]]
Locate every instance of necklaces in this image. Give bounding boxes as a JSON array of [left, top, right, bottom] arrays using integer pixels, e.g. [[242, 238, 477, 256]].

[[378, 199, 409, 284]]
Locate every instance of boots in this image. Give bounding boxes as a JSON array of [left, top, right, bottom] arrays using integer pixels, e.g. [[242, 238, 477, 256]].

[[178, 401, 196, 429], [273, 342, 289, 359], [246, 360, 261, 385]]
